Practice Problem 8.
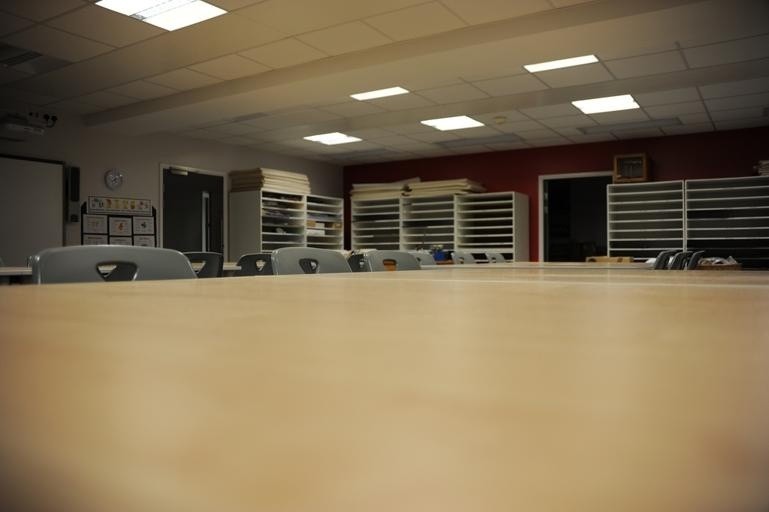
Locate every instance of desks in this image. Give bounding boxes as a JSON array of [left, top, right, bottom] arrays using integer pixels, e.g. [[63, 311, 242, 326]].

[[1, 262, 769, 512], [0, 261, 264, 283]]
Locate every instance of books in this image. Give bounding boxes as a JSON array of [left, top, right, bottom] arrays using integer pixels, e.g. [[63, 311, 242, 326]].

[[307, 223, 326, 236], [758, 160, 769, 176]]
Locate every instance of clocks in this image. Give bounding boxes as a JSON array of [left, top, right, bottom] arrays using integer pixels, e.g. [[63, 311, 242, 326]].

[[105, 170, 123, 190]]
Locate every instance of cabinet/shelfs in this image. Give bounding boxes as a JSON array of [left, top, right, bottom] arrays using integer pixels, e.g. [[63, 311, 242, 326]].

[[226, 188, 346, 261], [607, 180, 684, 260], [685, 176, 769, 266], [351, 190, 529, 263]]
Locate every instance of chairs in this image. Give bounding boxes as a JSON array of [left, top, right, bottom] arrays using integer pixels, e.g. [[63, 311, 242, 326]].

[[654, 251, 705, 270]]
